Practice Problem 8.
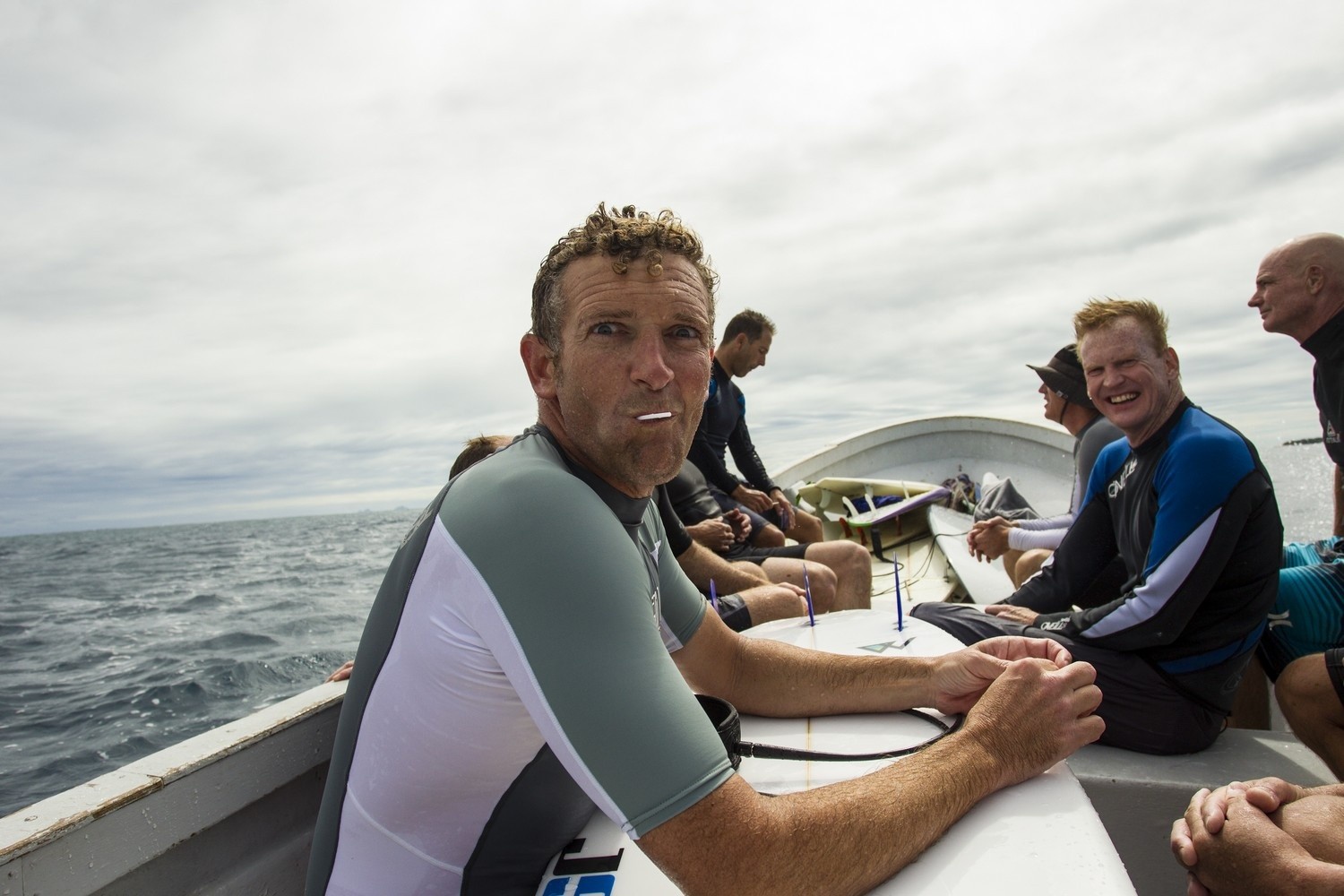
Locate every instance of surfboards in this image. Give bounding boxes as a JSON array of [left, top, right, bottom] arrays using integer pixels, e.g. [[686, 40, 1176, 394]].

[[535, 608, 1138, 896]]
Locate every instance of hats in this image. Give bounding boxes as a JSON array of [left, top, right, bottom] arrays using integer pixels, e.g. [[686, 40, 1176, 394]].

[[1025, 342, 1099, 411]]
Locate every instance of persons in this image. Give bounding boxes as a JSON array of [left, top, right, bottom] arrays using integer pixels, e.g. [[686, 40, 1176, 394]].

[[968, 344, 1125, 589], [907, 296, 1283, 756], [327, 435, 513, 680], [1249, 233, 1344, 680], [303, 203, 1104, 896], [650, 461, 871, 632], [686, 310, 824, 548], [1170, 647, 1344, 896]]
